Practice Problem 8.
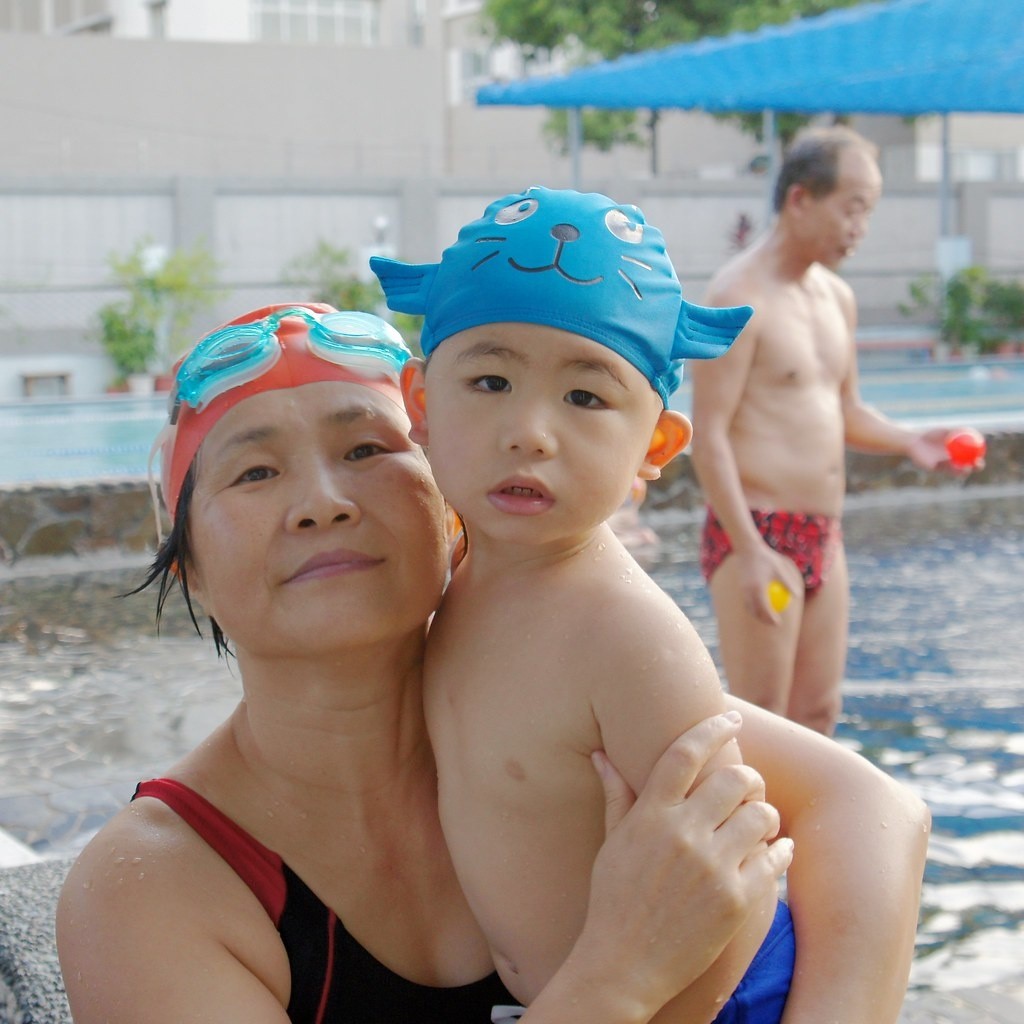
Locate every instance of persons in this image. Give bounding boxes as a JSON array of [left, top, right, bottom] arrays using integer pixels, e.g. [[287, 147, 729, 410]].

[[682, 124, 984, 738], [54, 186, 933, 1024]]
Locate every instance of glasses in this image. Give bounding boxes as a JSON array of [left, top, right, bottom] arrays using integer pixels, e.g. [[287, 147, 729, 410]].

[[147, 311, 415, 545]]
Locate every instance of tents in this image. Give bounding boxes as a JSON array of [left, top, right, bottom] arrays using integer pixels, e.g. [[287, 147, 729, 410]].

[[478, 3, 1022, 333]]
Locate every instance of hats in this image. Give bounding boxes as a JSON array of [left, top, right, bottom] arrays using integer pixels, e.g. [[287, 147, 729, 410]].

[[157, 303, 402, 524], [370, 187, 755, 410]]
